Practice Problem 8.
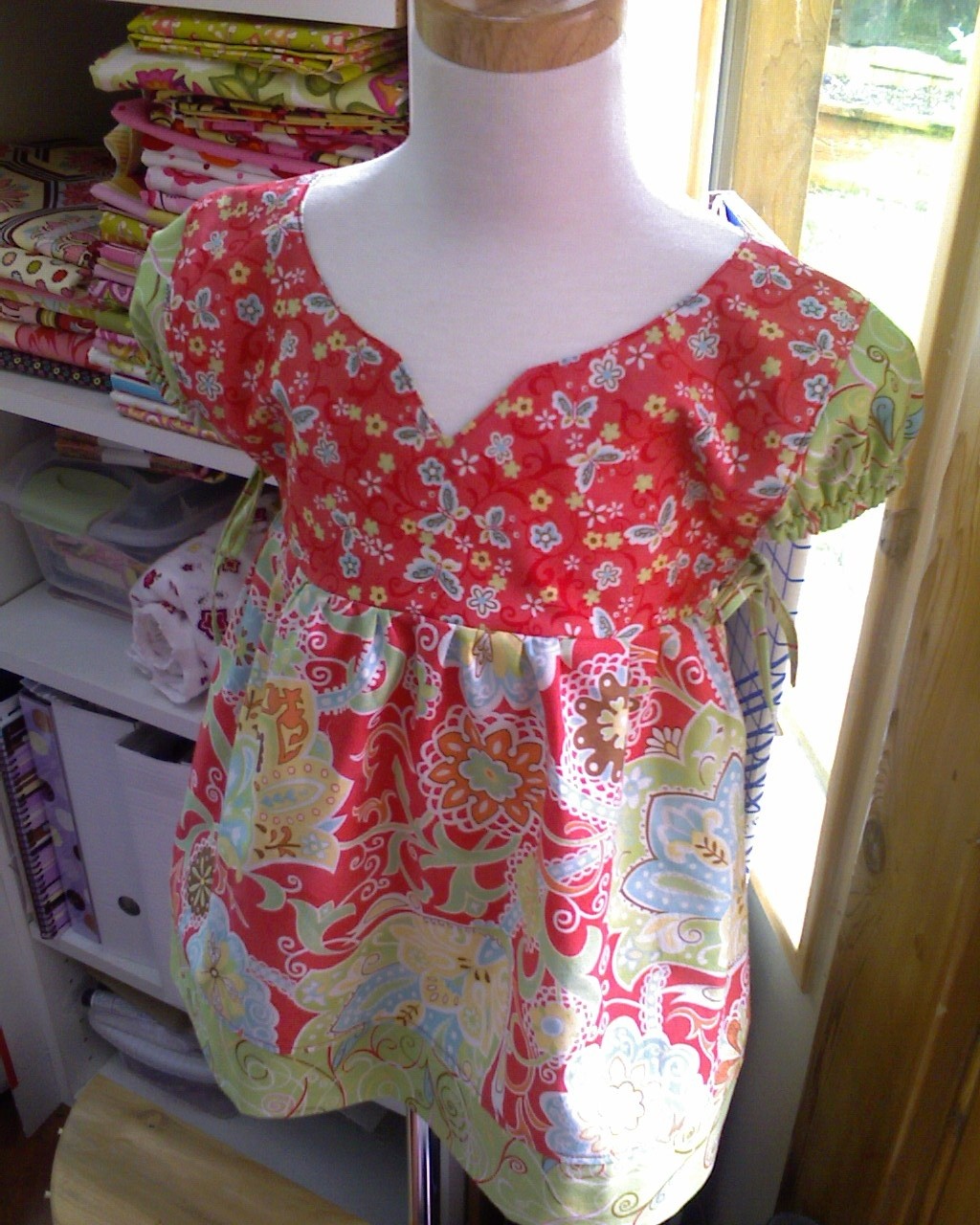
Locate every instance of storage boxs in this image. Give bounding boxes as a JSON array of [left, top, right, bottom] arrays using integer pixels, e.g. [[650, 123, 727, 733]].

[[0, 436, 237, 616]]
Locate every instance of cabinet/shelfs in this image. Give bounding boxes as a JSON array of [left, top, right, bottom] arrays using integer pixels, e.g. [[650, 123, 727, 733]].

[[0, 0, 462, 1225]]
